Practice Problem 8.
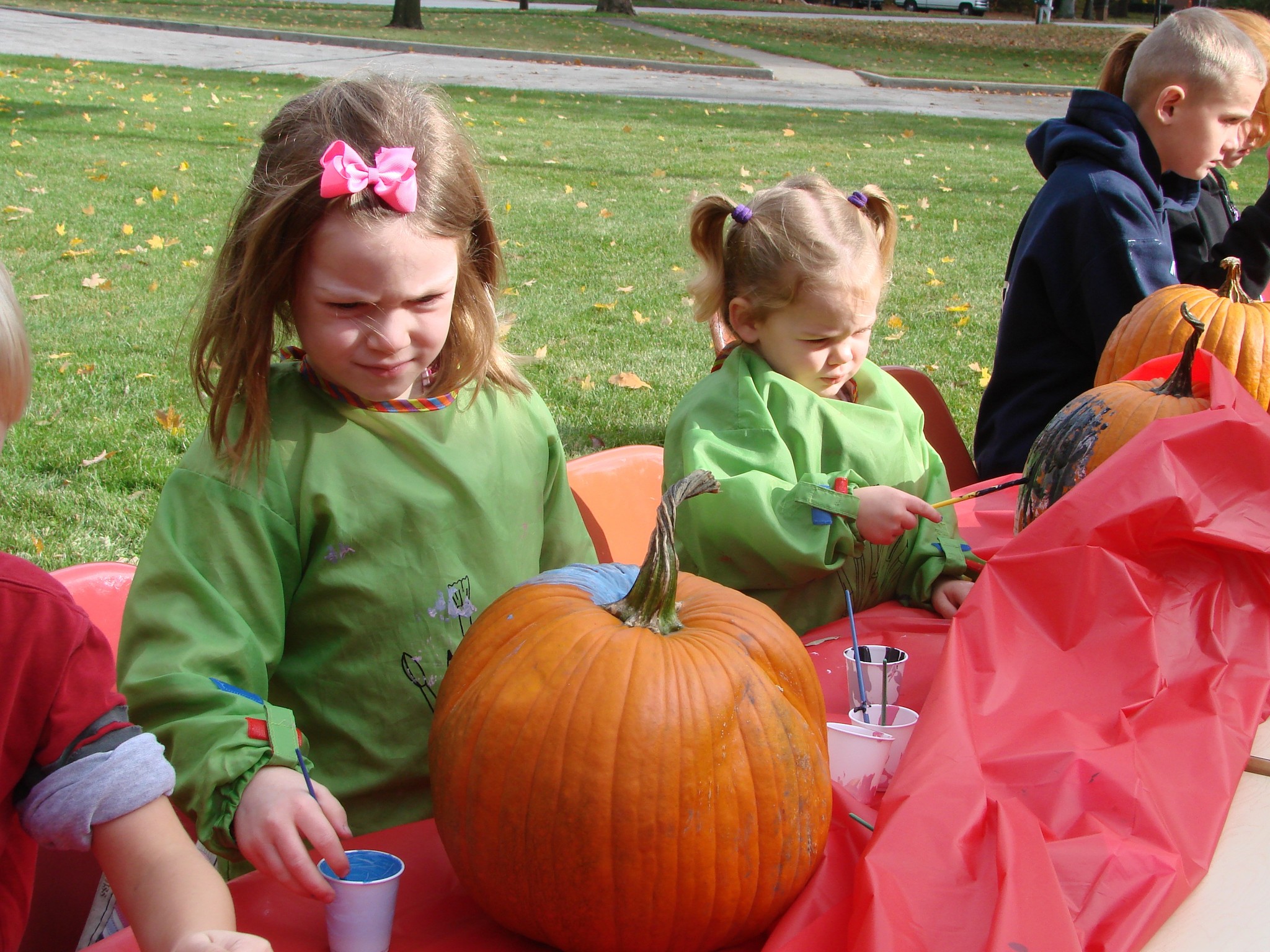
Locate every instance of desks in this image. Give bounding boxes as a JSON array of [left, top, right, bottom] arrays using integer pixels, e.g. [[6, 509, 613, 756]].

[[84, 345, 1270, 952]]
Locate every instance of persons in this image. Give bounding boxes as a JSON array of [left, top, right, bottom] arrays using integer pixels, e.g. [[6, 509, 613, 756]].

[[0, 259, 271, 952], [662, 175, 985, 636], [974, 6, 1270, 479], [76, 67, 602, 952], [1098, 8, 1270, 305]]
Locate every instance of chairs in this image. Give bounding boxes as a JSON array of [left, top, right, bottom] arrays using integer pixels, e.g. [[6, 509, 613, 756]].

[[49, 561, 137, 666], [567, 445, 663, 567], [880, 365, 979, 493]]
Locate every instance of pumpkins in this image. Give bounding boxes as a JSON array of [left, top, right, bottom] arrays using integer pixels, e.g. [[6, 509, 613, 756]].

[[1012, 301, 1211, 539], [430, 470, 832, 951], [1094, 257, 1270, 413]]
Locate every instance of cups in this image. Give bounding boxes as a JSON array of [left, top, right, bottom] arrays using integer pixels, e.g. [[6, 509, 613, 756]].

[[851, 704, 920, 789], [842, 645, 909, 706], [827, 721, 895, 807], [317, 848, 404, 952]]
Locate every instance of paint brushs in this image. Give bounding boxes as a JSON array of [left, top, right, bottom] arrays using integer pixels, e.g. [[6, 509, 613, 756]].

[[930, 477, 1029, 509], [881, 658, 887, 725], [846, 589, 869, 724], [295, 747, 347, 881]]
